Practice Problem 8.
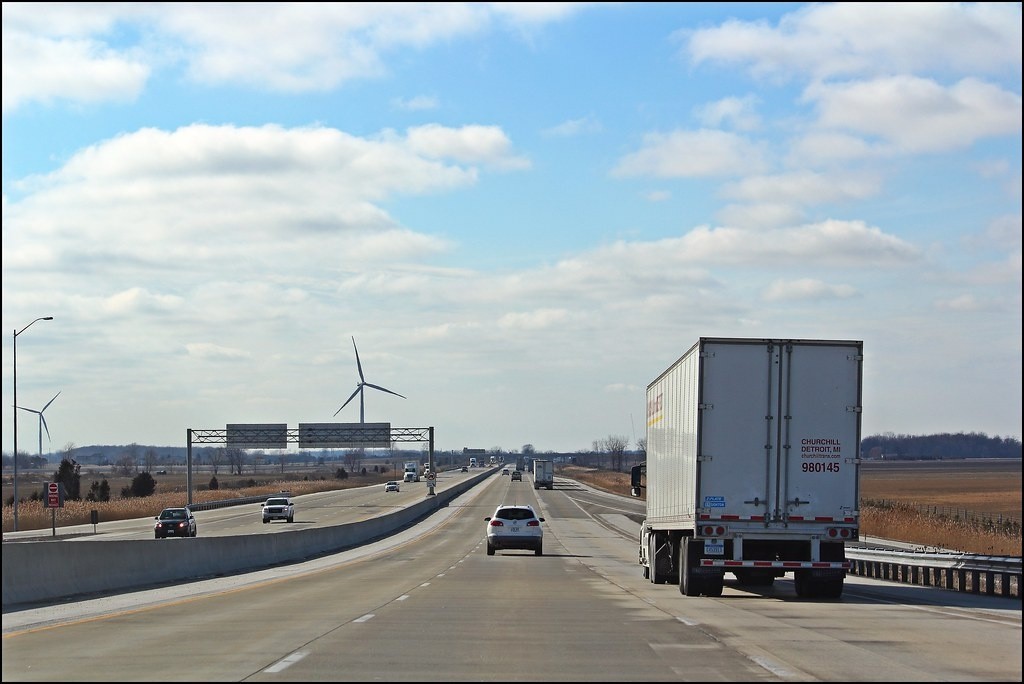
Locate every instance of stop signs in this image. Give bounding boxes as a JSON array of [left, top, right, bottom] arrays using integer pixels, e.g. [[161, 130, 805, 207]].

[[48, 483, 59, 494]]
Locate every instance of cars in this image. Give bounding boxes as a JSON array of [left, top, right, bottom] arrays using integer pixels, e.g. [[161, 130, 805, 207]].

[[501, 469, 510, 476], [511, 471, 522, 482], [461, 466, 468, 473], [384, 481, 400, 492]]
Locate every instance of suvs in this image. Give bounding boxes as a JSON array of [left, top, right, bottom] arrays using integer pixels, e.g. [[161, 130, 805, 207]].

[[261, 498, 295, 523], [484, 504, 545, 556], [154, 506, 196, 538]]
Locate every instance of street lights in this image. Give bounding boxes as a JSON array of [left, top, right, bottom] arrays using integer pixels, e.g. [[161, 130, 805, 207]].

[[14, 317, 53, 532]]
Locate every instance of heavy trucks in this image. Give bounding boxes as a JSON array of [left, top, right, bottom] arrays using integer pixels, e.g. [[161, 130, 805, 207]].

[[630, 336, 863, 599]]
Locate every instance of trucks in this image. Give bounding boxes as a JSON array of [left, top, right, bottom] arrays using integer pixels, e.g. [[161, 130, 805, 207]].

[[403, 460, 421, 482], [470, 458, 476, 467], [528, 458, 539, 473], [479, 460, 485, 468], [532, 460, 553, 490], [490, 456, 497, 464], [424, 462, 436, 478], [516, 457, 526, 471]]
